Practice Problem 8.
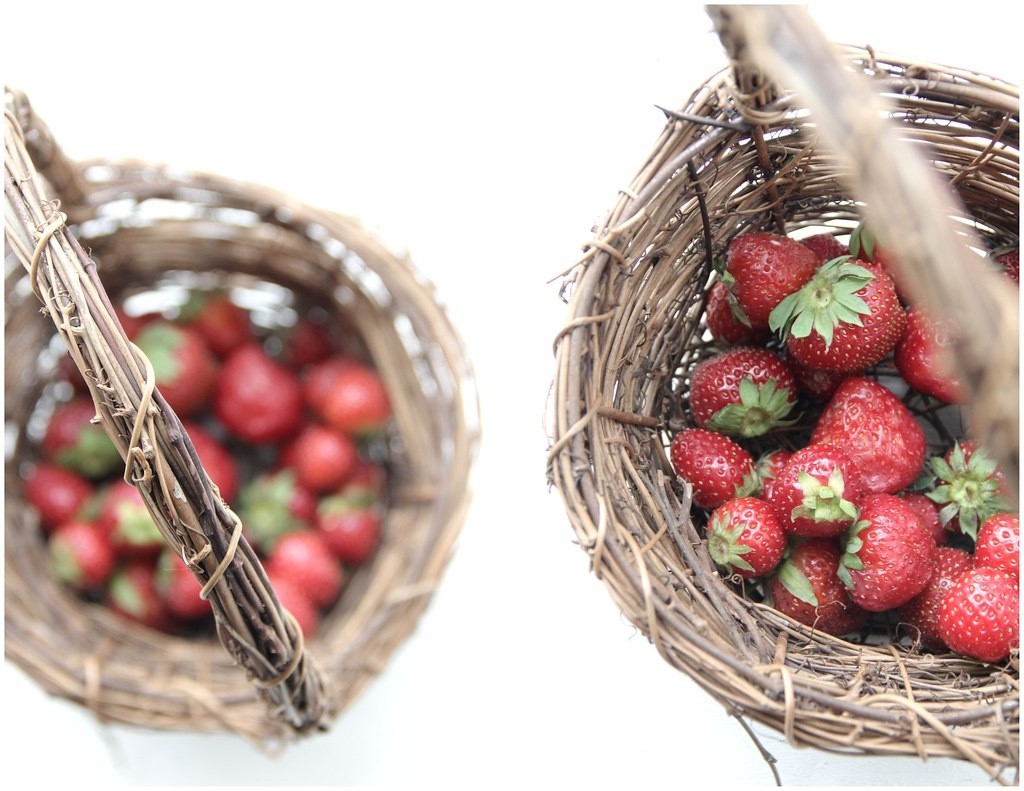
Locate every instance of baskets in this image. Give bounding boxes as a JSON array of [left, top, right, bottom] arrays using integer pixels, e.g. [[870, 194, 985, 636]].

[[4, 87, 479, 759], [548, 5, 1019, 785]]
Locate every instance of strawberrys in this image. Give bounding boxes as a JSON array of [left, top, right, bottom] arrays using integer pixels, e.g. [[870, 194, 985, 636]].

[[22, 285, 395, 641], [669, 220, 1019, 663]]
